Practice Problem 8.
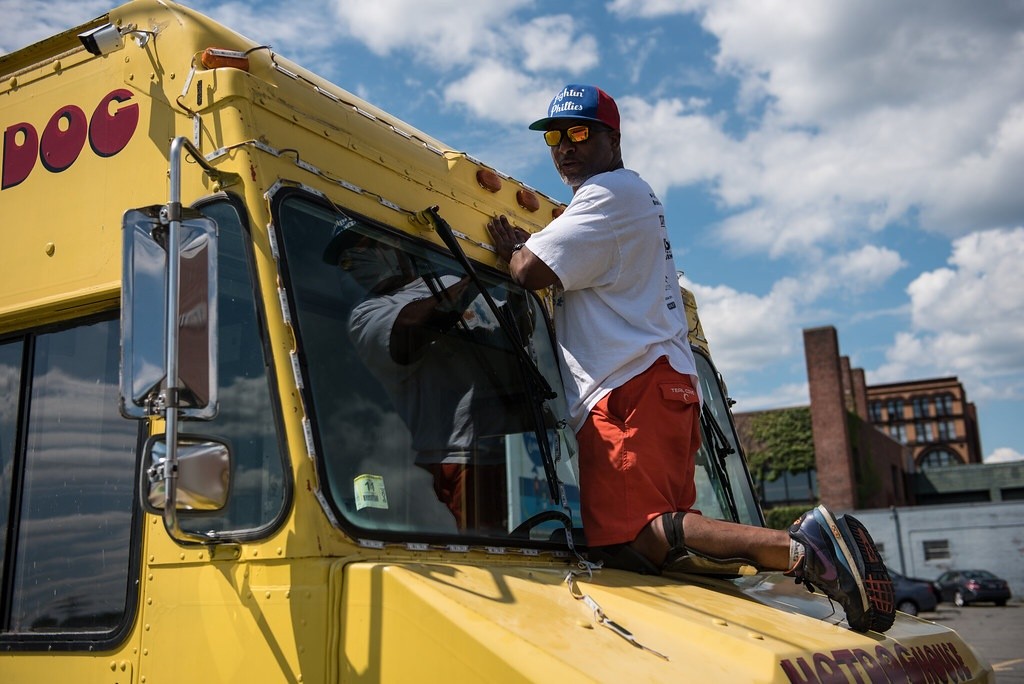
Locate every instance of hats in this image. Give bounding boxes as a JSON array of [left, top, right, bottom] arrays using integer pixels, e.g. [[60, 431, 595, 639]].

[[529, 84, 620, 132], [323, 210, 368, 265]]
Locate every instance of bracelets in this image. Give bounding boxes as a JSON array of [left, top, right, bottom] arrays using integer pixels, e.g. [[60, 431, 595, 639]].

[[513, 240, 525, 249]]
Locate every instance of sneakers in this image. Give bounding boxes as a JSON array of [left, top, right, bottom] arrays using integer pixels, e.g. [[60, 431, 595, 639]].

[[837, 513, 896, 633], [787, 504, 876, 633]]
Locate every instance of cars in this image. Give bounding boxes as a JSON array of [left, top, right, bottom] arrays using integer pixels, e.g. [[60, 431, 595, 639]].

[[885, 566, 941, 618], [932, 569, 1012, 607]]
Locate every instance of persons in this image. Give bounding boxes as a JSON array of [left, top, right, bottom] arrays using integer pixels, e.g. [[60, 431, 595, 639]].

[[326, 232, 578, 535], [487, 85, 897, 632]]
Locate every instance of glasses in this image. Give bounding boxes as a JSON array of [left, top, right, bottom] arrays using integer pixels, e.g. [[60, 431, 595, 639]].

[[338, 236, 370, 271], [544, 124, 613, 147]]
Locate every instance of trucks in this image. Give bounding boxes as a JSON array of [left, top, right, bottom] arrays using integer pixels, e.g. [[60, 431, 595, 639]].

[[1, 1, 993, 684]]
